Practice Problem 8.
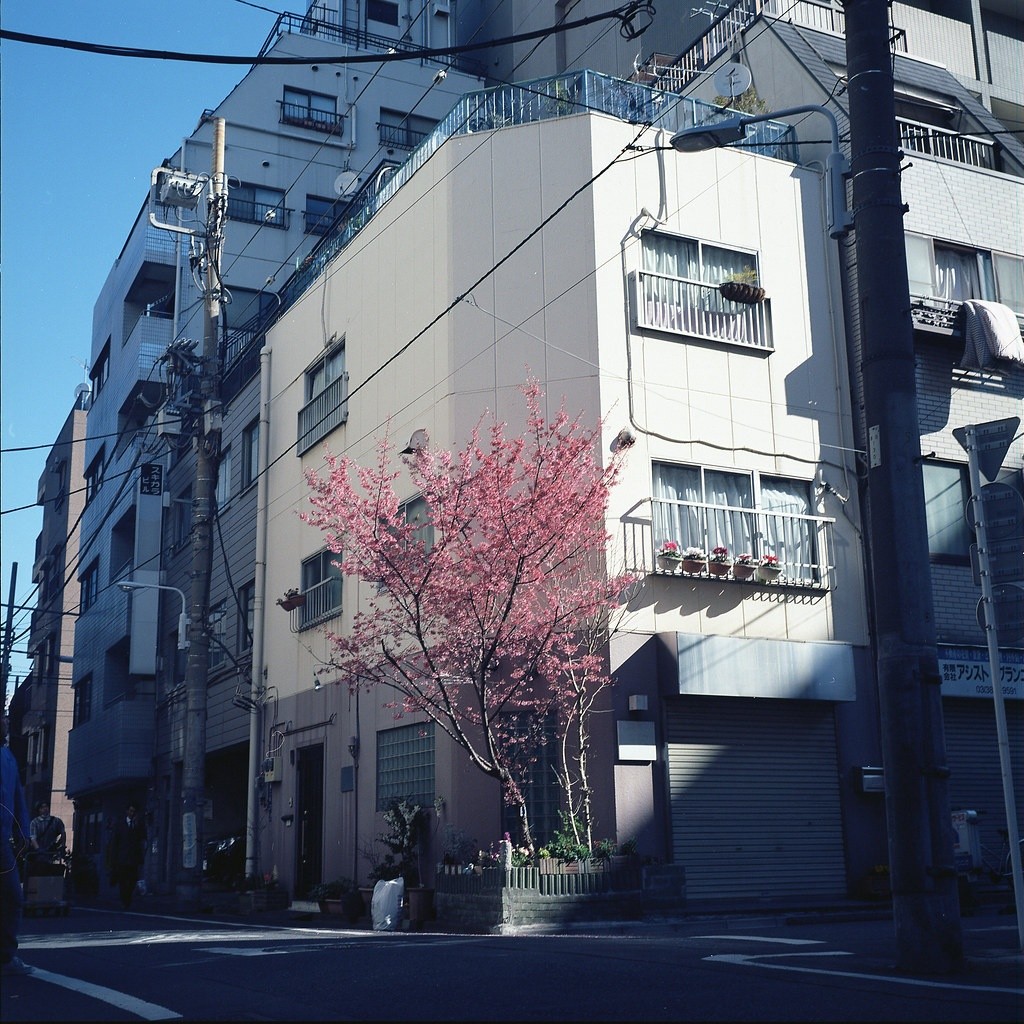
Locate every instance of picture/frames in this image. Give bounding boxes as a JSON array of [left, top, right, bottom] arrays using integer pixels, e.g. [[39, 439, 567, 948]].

[[251, 890, 289, 913]]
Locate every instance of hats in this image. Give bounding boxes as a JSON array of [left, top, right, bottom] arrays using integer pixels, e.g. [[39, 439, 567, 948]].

[[34, 800, 49, 811]]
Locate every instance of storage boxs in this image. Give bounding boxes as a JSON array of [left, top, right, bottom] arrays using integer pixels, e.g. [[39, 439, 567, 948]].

[[27, 876, 63, 902]]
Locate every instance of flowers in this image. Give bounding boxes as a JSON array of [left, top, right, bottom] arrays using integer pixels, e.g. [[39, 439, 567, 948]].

[[865, 864, 890, 880], [378, 795, 445, 887], [232, 872, 255, 893], [733, 553, 754, 564], [284, 588, 298, 599], [709, 546, 728, 563], [264, 873, 273, 889], [682, 545, 707, 561], [656, 542, 682, 558], [759, 554, 781, 567]]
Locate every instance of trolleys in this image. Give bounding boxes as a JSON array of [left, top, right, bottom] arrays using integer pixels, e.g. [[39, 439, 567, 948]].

[[22, 850, 71, 918]]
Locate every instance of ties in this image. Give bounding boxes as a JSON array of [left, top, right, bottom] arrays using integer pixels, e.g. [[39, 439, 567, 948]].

[[129, 820, 133, 830]]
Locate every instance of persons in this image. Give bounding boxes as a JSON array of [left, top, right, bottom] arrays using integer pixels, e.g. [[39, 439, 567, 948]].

[[31, 801, 66, 915], [113, 803, 145, 909], [0, 707, 30, 974]]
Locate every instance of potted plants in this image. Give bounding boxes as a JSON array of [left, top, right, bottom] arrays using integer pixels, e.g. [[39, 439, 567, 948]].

[[719, 266, 765, 304], [276, 599, 296, 612], [357, 855, 410, 919], [311, 879, 360, 914]]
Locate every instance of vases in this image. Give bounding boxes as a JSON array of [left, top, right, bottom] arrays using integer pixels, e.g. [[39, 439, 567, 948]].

[[732, 564, 756, 578], [657, 556, 682, 570], [707, 560, 731, 576], [289, 595, 304, 607], [680, 559, 706, 573], [758, 567, 783, 580], [409, 888, 435, 931], [238, 894, 251, 912]]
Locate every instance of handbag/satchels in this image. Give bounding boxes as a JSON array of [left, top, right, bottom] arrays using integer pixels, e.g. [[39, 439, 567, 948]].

[[109, 866, 118, 887]]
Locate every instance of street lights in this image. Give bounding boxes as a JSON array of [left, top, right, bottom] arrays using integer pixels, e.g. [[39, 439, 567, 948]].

[[115, 580, 205, 913], [671, 101, 961, 974]]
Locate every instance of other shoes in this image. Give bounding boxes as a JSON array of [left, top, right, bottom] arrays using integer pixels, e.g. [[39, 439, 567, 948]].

[[0, 956, 34, 976], [120, 902, 128, 910]]
